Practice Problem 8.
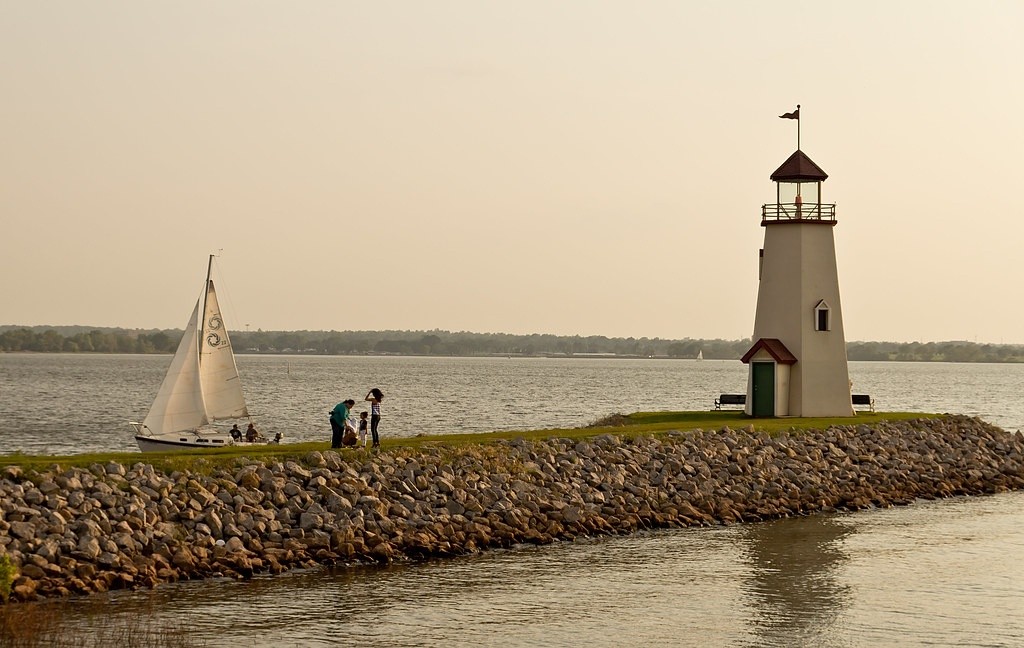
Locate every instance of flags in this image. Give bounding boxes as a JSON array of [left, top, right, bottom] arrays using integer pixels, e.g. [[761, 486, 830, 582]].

[[779, 109, 799, 120]]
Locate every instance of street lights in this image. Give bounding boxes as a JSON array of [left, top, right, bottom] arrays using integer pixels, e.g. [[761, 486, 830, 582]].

[[246, 324, 250, 332]]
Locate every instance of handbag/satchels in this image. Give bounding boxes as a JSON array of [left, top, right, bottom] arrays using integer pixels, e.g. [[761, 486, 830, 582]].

[[343, 426, 357, 445]]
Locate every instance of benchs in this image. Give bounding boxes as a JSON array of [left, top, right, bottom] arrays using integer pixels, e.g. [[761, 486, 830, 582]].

[[852, 394, 874, 412], [714, 394, 745, 410]]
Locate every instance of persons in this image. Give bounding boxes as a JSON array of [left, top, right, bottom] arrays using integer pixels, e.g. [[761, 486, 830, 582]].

[[246, 424, 258, 442], [355, 412, 369, 450], [330, 399, 355, 448], [229, 424, 242, 442], [365, 388, 384, 448]]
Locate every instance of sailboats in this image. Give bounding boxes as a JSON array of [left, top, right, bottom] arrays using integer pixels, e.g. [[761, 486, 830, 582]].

[[127, 254, 283, 452]]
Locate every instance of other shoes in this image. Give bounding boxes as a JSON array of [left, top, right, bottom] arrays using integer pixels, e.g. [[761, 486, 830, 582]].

[[371, 443, 380, 448]]
